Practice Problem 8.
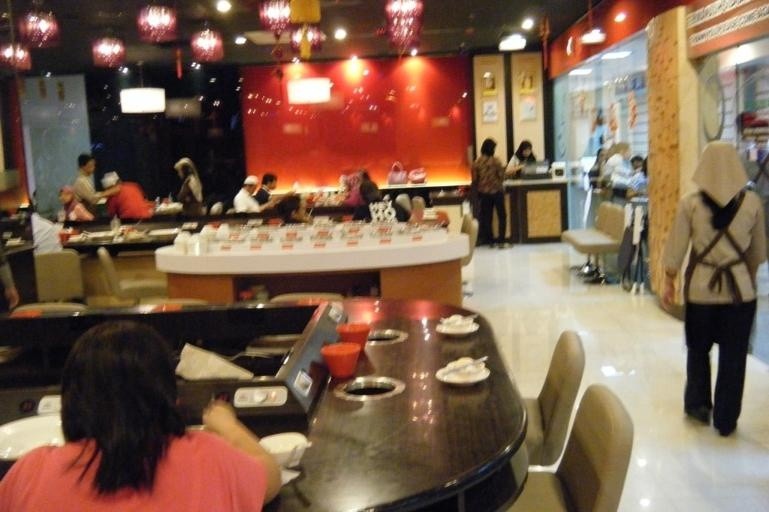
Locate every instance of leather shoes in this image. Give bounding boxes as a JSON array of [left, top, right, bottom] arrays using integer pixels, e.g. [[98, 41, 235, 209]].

[[717, 422, 737, 437], [686, 405, 710, 426]]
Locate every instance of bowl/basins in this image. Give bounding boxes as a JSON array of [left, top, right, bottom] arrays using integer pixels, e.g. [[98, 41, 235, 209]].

[[334, 322, 370, 353], [258, 431, 313, 468]]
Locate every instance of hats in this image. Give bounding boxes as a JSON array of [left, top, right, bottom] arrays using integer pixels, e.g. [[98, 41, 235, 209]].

[[244, 175, 258, 185]]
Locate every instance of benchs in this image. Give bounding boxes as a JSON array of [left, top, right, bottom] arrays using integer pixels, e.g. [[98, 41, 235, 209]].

[[560, 200, 625, 288]]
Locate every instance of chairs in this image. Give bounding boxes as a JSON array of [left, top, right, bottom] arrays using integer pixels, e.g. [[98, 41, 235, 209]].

[[508, 383, 634, 512], [520, 331, 585, 469]]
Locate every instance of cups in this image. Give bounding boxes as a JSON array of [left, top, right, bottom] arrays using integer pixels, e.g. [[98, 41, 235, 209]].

[[320, 341, 362, 379]]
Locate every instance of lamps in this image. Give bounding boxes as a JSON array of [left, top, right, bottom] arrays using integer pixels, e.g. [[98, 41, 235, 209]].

[[291, 1, 322, 60], [258, 0, 291, 60], [285, 63, 333, 104], [15, 0, 62, 48], [89, 27, 125, 69], [120, 61, 166, 114], [380, 1, 424, 57], [293, 24, 322, 56], [1, 1, 31, 74], [135, 0, 180, 45], [188, 21, 224, 63]]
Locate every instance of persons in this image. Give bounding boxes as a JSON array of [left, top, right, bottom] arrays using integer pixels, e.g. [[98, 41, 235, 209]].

[[734, 110, 757, 155], [741, 120, 769, 210], [471, 139, 514, 250], [0, 319, 282, 512], [505, 140, 536, 176], [73, 153, 121, 220], [58, 185, 96, 221], [352, 179, 411, 222], [585, 148, 608, 227], [658, 142, 766, 437], [618, 155, 647, 293], [174, 157, 203, 219], [0, 242, 20, 311], [254, 173, 277, 205], [232, 175, 260, 213], [337, 174, 367, 207], [101, 171, 155, 220], [273, 194, 315, 226], [603, 141, 634, 206], [629, 150, 644, 174]]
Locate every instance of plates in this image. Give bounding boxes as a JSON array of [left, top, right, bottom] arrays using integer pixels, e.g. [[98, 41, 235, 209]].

[[0, 413, 67, 462], [436, 322, 480, 339], [436, 360, 491, 387]]
